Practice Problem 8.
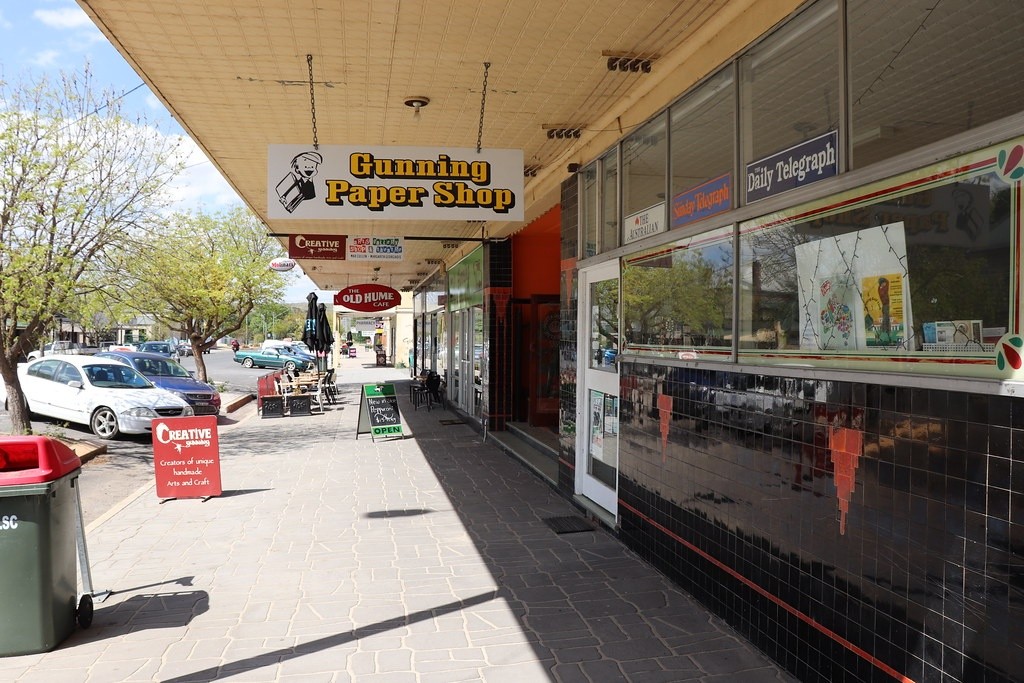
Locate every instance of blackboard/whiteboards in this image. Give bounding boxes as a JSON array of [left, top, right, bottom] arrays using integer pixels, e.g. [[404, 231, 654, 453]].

[[366, 395, 400, 426]]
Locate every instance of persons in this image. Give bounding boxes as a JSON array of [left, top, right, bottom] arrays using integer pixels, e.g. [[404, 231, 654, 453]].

[[231, 340, 239, 351]]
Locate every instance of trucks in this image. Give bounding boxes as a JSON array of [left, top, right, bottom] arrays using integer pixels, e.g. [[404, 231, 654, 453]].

[[25, 340, 105, 364]]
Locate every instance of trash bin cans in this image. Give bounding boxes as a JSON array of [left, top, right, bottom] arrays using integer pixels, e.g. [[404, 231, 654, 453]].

[[0, 436, 95, 657]]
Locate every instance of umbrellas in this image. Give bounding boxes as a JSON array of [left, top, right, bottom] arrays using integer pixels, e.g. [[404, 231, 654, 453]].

[[300, 292, 335, 379], [346, 331, 353, 346]]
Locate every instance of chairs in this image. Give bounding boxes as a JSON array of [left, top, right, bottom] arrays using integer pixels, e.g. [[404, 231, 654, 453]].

[[273, 368, 339, 413], [136, 360, 147, 372], [414, 370, 443, 413], [95, 370, 108, 381]]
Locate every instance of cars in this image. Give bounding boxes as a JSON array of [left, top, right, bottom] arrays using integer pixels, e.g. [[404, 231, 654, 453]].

[[0, 355, 195, 441], [177, 344, 197, 358], [133, 341, 181, 373], [232, 339, 317, 374], [94, 350, 222, 421]]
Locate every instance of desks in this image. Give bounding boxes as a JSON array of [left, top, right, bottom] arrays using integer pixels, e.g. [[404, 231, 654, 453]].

[[409, 380, 428, 404]]
